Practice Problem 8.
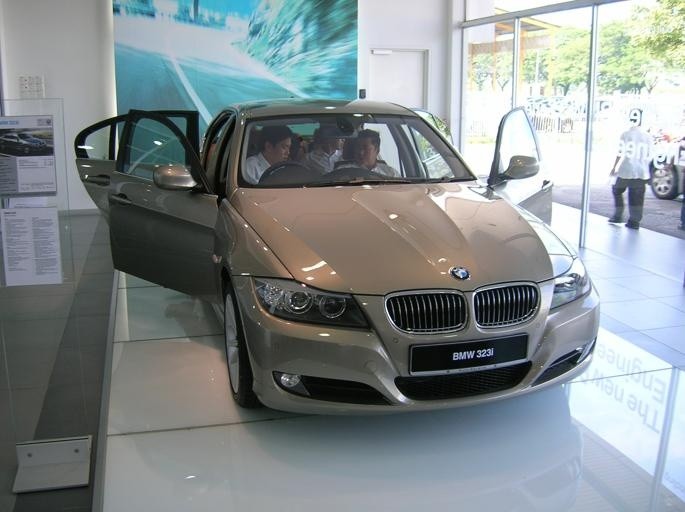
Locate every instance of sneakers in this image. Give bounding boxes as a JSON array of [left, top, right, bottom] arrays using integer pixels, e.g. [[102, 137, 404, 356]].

[[607, 214, 623, 224], [625, 217, 640, 230], [677, 221, 685, 230]]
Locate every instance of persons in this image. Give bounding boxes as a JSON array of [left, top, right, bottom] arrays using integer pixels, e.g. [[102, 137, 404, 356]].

[[247, 122, 403, 184], [607, 109, 655, 229], [678, 169, 685, 230]]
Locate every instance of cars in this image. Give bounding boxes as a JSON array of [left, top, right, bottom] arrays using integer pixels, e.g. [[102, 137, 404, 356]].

[[0, 133, 47, 154], [575, 99, 610, 114], [524, 98, 581, 133], [73, 97, 602, 416], [647, 134, 684, 199]]
